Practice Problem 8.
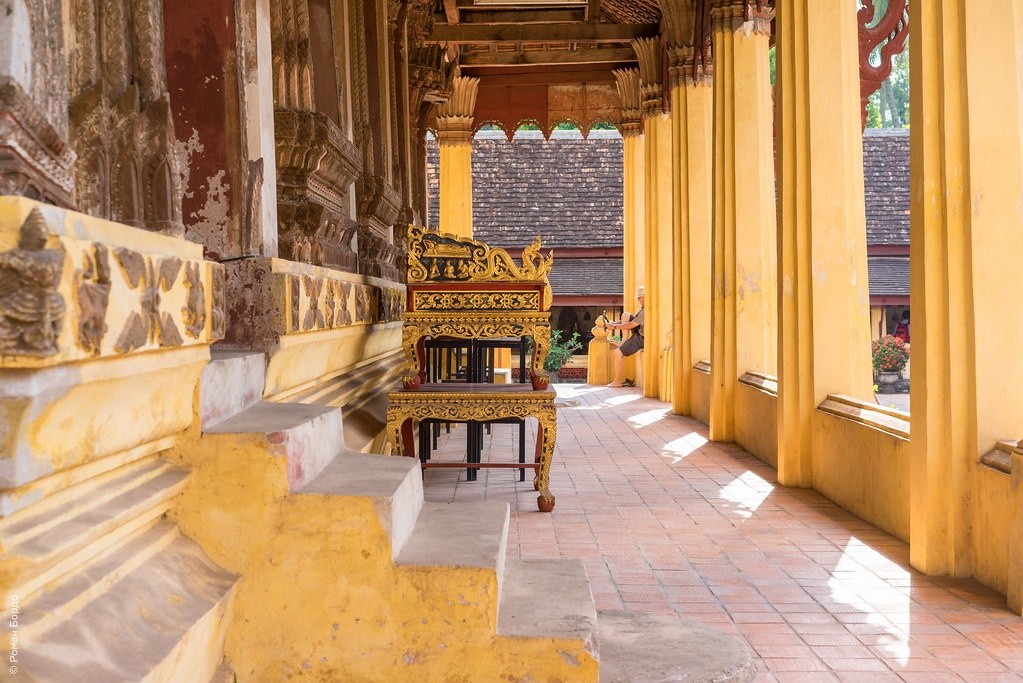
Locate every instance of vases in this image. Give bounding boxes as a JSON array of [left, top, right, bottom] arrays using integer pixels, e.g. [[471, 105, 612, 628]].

[[877, 372, 901, 384]]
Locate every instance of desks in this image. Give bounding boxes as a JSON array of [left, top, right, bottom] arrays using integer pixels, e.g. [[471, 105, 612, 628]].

[[385, 381, 557, 512]]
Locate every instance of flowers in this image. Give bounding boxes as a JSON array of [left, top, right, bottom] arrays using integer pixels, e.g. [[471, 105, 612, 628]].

[[871, 334, 910, 371]]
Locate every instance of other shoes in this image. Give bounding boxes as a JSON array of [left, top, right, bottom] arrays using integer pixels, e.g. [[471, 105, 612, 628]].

[[603, 314, 610, 333], [621, 378, 635, 387]]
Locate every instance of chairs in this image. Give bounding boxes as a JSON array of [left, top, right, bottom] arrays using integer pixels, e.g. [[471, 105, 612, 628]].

[[400, 224, 553, 391], [420, 335, 526, 483]]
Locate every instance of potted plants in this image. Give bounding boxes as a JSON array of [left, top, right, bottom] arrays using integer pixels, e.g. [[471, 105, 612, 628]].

[[527, 330, 583, 381]]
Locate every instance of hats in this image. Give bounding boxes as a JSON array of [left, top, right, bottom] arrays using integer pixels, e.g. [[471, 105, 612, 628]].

[[634, 286, 645, 298]]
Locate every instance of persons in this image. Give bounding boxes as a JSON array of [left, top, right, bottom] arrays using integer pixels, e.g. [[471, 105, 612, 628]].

[[893, 310, 910, 343], [605, 286, 644, 387]]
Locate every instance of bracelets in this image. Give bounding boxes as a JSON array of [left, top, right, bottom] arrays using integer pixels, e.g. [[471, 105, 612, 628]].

[[614, 324, 616, 329]]
